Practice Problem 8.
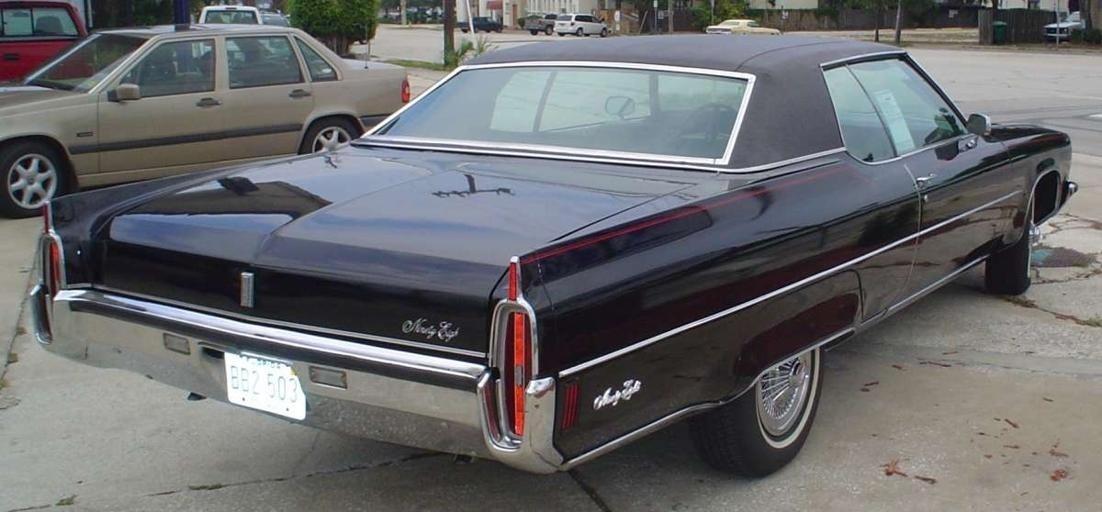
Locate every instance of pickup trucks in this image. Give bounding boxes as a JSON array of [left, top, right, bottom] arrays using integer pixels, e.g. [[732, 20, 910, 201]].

[[457, 17, 504, 33], [525, 14, 557, 36]]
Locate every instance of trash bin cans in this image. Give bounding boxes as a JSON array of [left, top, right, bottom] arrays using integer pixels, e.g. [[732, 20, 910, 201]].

[[993, 20, 1008, 43]]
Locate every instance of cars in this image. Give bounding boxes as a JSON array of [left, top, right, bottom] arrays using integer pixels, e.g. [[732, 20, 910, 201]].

[[0, 22, 412, 221], [1, 0, 98, 88], [705, 18, 782, 40], [1041, 11, 1086, 45], [195, 0, 294, 64], [28, 34, 1079, 479]]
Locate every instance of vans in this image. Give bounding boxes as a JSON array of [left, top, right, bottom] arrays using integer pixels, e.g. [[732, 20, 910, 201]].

[[553, 13, 609, 37]]
